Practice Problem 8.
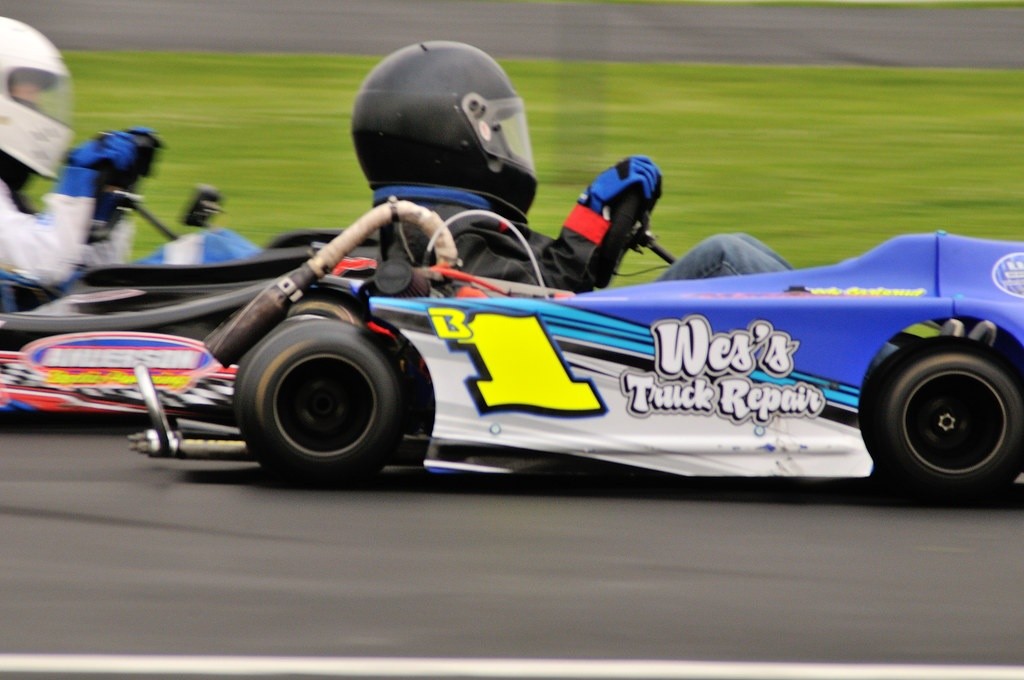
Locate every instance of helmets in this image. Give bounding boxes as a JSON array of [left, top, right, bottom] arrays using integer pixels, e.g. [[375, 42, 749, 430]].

[[351, 39, 537, 224], [0, 15, 74, 182]]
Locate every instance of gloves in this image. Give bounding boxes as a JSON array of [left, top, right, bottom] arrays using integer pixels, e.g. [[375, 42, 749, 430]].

[[68, 124, 161, 187], [577, 155, 663, 215]]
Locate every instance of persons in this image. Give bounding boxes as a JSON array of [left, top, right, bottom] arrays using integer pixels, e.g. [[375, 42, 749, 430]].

[[352, 39, 793, 296], [0, 17, 266, 314]]
